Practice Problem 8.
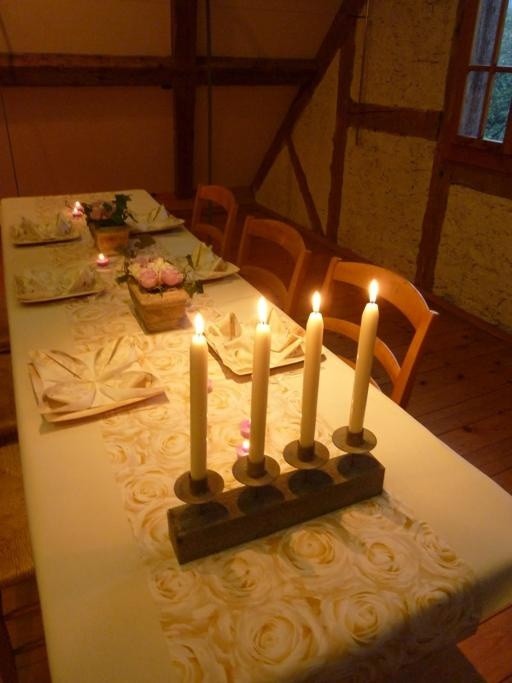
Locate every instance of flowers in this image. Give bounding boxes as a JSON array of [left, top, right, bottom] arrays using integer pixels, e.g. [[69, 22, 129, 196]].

[[89, 199, 115, 222], [126, 252, 186, 289]]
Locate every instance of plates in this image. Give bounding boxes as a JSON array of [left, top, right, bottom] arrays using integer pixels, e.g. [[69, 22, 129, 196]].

[[23, 336, 169, 425], [166, 252, 242, 283], [8, 221, 82, 247], [183, 294, 326, 378], [12, 260, 109, 306], [126, 209, 186, 236]]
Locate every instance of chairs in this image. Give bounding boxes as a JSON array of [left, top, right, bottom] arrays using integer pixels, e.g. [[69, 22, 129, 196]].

[[237, 214, 313, 314], [191, 184, 239, 257], [0, 352, 18, 440], [0, 444, 50, 682], [318, 255, 439, 409]]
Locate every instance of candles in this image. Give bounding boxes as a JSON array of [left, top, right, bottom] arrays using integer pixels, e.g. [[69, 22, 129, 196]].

[[189, 313, 208, 494], [246, 295, 271, 477], [298, 291, 324, 461], [347, 278, 379, 444]]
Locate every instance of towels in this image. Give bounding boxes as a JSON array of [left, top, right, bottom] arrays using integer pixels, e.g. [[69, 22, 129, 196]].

[[15, 262, 103, 300], [27, 334, 169, 416], [123, 205, 180, 231], [12, 210, 75, 241], [165, 241, 230, 282], [199, 299, 322, 371]]
